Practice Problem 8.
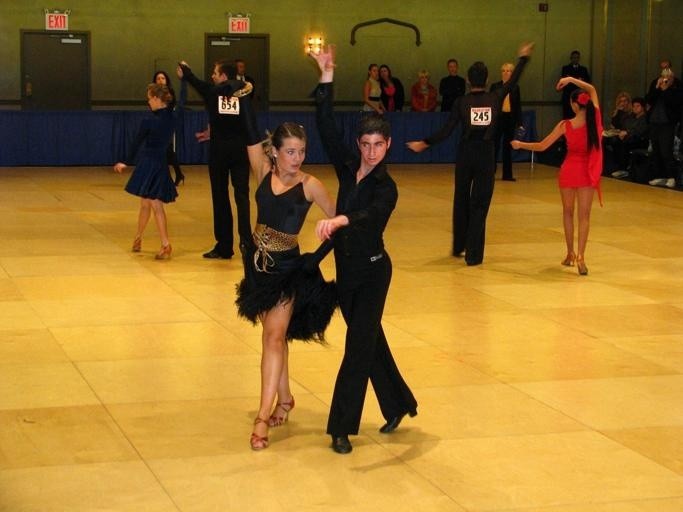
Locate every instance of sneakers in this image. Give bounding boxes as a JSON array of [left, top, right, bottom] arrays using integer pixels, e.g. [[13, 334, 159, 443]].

[[649, 178, 676, 188], [606, 165, 629, 179]]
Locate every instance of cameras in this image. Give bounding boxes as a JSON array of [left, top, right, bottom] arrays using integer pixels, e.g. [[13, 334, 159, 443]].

[[663, 78, 669, 84]]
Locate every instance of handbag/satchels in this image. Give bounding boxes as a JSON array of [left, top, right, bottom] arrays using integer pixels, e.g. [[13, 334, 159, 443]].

[[602, 128, 620, 137]]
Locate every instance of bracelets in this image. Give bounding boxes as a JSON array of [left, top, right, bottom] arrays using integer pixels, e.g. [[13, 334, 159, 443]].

[[232, 82, 252, 96]]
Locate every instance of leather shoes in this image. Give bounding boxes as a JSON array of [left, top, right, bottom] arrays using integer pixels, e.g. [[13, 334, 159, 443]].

[[502, 177, 516, 181], [330, 433, 352, 453], [380, 406, 417, 432], [203, 244, 246, 259], [453, 245, 482, 265]]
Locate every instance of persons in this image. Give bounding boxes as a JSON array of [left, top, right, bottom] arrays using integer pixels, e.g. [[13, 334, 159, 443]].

[[509, 76, 604, 274], [112, 65, 187, 259], [405, 41, 535, 266], [179, 60, 254, 259], [216, 78, 336, 450], [308, 44, 418, 453], [643, 68, 683, 189], [153, 50, 645, 181]]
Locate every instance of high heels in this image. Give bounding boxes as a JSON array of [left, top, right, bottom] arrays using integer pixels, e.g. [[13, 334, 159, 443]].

[[561, 250, 576, 265], [156, 245, 172, 259], [250, 415, 270, 450], [576, 256, 588, 275], [267, 396, 294, 427], [175, 174, 184, 186], [133, 235, 141, 252]]
